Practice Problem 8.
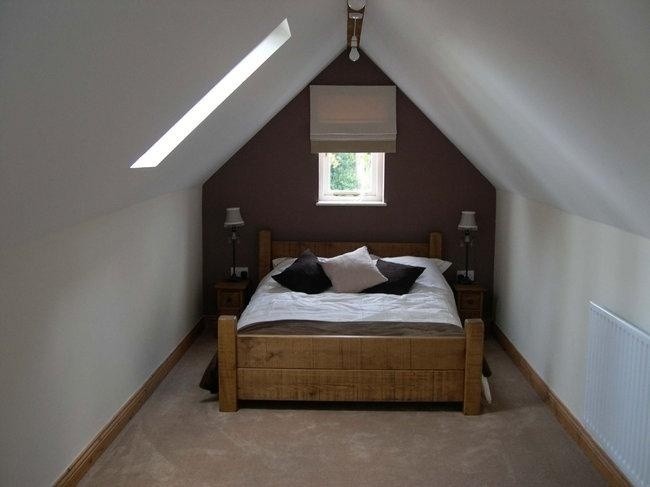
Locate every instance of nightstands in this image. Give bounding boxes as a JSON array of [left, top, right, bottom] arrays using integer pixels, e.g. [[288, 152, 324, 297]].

[[214, 281, 248, 340], [456, 284, 486, 318]]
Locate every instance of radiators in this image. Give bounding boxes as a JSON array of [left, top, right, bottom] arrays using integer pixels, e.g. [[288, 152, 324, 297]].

[[584, 299, 650, 486]]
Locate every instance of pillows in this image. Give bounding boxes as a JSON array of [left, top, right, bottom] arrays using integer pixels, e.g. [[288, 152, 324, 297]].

[[362, 259, 426, 294], [316, 246, 388, 293], [271, 249, 333, 294]]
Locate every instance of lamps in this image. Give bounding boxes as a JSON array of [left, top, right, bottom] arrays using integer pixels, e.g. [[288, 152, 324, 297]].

[[458, 211, 477, 285], [224, 208, 244, 283], [349, 13, 363, 61]]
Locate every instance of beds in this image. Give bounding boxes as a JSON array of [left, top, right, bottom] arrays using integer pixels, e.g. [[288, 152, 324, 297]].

[[218, 230, 492, 416]]
